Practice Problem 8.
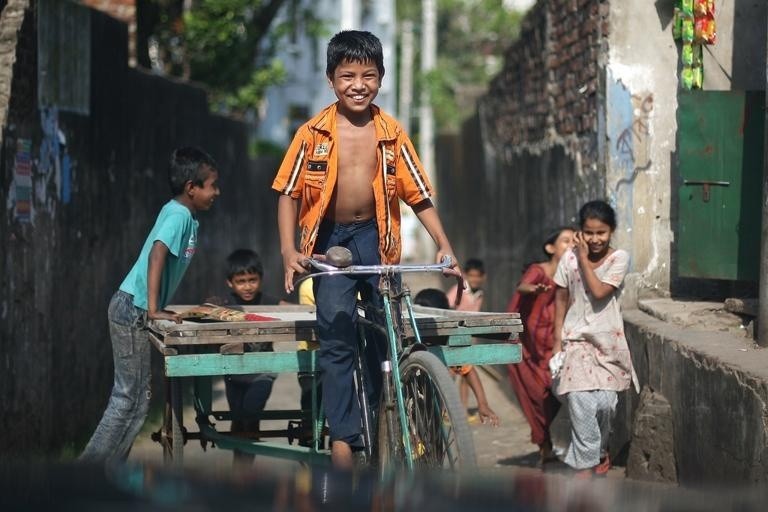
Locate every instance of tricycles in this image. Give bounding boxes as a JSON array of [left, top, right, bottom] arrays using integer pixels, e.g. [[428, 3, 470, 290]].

[[150, 246, 525, 508]]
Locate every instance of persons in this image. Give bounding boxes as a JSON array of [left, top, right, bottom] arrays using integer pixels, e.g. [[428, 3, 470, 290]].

[[294, 276, 363, 411], [74, 143, 221, 466], [501, 228, 580, 463], [413, 288, 500, 429], [272, 29, 460, 474], [201, 247, 295, 469], [446, 258, 484, 422], [546, 200, 634, 482]]
